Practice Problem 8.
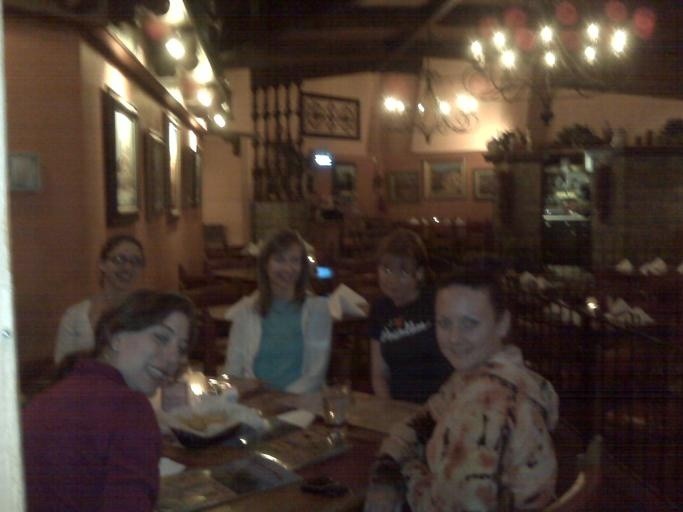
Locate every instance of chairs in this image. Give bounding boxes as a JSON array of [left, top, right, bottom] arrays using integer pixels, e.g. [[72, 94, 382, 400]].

[[171, 252, 391, 390], [528, 419, 602, 512]]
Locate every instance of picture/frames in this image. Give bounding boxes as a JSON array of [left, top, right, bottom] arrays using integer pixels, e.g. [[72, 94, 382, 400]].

[[330, 155, 496, 202], [104, 82, 203, 227]]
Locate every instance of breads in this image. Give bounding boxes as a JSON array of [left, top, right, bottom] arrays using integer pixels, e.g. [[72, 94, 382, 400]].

[[174, 408, 228, 432]]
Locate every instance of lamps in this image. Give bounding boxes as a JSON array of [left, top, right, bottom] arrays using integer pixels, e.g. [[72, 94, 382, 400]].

[[383, 0, 628, 145]]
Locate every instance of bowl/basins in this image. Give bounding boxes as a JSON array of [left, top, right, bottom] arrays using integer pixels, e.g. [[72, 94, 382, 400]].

[[165, 399, 250, 441]]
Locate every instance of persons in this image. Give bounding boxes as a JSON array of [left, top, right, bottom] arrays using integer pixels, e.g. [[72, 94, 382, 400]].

[[18, 290, 202, 511], [220, 226, 337, 395], [367, 267, 563, 511], [52, 235, 146, 372], [369, 229, 454, 406]]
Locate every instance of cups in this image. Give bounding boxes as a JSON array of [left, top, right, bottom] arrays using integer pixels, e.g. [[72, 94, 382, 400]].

[[321, 377, 352, 426]]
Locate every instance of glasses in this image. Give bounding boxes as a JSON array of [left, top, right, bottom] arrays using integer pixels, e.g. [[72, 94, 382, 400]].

[[110, 255, 141, 267]]
